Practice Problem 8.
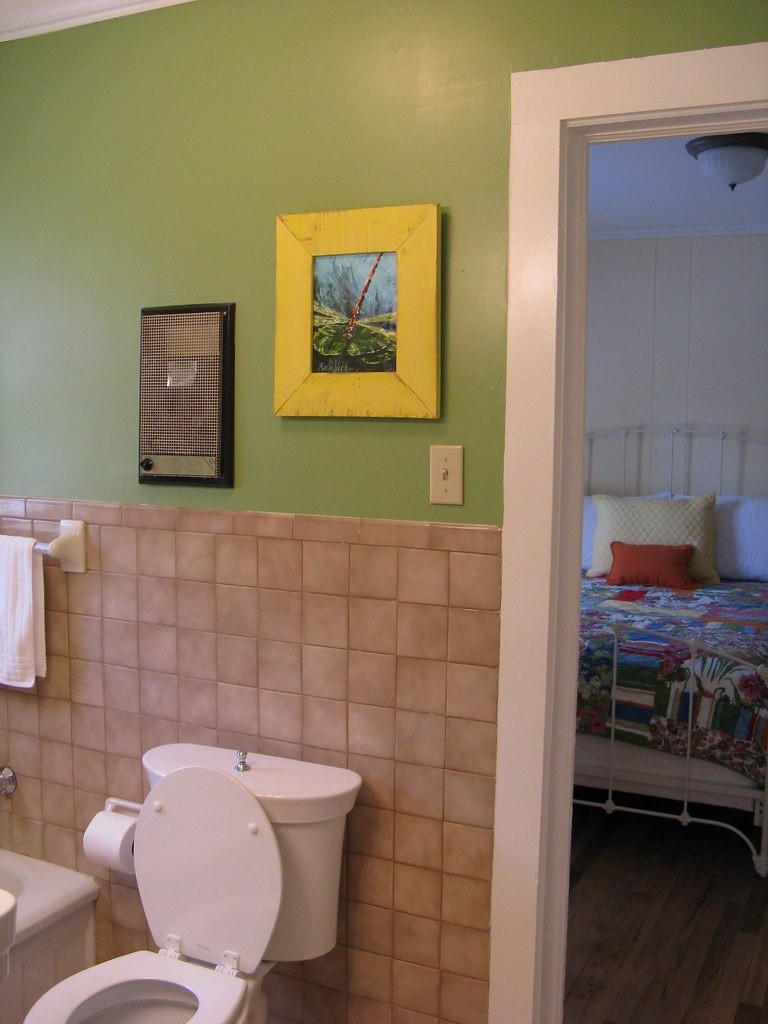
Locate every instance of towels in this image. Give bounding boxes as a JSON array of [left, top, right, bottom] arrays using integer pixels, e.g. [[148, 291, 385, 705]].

[[0, 534, 46, 688]]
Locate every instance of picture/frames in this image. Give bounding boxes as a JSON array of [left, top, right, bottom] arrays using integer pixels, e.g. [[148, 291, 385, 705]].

[[271, 201, 444, 418]]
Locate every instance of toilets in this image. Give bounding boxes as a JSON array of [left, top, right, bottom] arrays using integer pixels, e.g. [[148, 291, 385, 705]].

[[22, 744, 364, 1024]]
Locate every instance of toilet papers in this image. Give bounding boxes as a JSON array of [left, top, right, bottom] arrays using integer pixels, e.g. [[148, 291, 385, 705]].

[[83, 810, 137, 876]]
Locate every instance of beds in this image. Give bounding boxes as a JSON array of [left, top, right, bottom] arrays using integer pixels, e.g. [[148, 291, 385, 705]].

[[573, 423, 768, 877]]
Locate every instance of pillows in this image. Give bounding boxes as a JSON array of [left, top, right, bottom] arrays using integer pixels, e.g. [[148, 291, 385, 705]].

[[606, 540, 695, 590], [671, 494, 768, 583], [582, 492, 671, 582], [586, 491, 720, 584]]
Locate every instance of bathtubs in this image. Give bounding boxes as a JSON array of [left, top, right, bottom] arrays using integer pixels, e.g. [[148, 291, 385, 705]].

[[1, 847, 99, 1024]]
